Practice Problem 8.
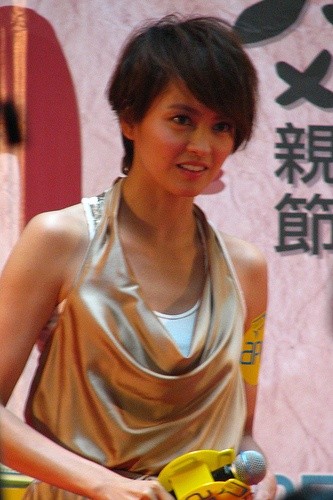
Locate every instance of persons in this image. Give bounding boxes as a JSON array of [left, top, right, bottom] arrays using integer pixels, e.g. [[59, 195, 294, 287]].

[[0, 16, 277, 500]]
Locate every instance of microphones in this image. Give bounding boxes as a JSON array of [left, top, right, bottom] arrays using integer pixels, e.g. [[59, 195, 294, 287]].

[[167, 451, 267, 499]]
